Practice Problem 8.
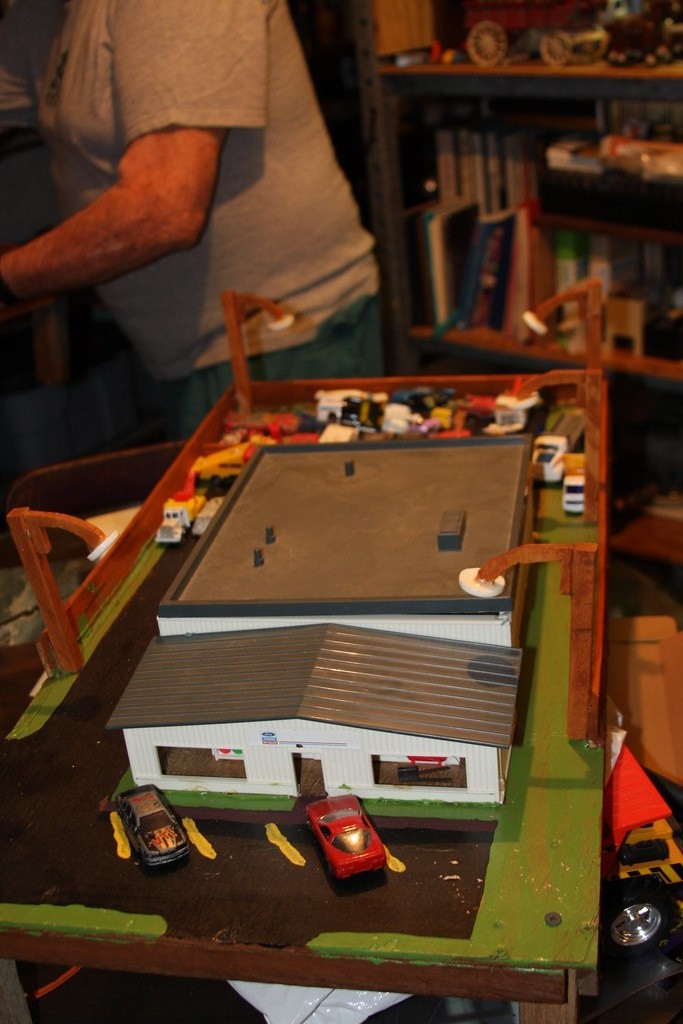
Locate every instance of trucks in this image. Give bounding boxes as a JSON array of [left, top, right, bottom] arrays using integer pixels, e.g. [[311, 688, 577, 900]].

[[155, 495, 205, 547], [531, 410, 584, 487], [561, 453, 585, 515]]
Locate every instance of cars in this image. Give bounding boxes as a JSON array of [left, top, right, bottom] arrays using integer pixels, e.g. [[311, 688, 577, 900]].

[[599, 816, 683, 960], [114, 783, 191, 870], [305, 795, 386, 881]]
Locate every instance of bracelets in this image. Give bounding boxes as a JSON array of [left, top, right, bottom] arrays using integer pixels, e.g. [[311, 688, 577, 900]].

[[0, 276, 22, 306]]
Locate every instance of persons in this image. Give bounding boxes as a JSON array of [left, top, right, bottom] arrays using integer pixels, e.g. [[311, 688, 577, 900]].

[[0, 0, 381, 434]]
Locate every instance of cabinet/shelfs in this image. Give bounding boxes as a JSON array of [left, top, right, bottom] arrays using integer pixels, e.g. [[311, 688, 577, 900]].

[[352, 0, 683, 560]]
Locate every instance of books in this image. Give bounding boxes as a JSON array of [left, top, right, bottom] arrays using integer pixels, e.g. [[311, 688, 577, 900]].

[[409, 117, 540, 334]]
